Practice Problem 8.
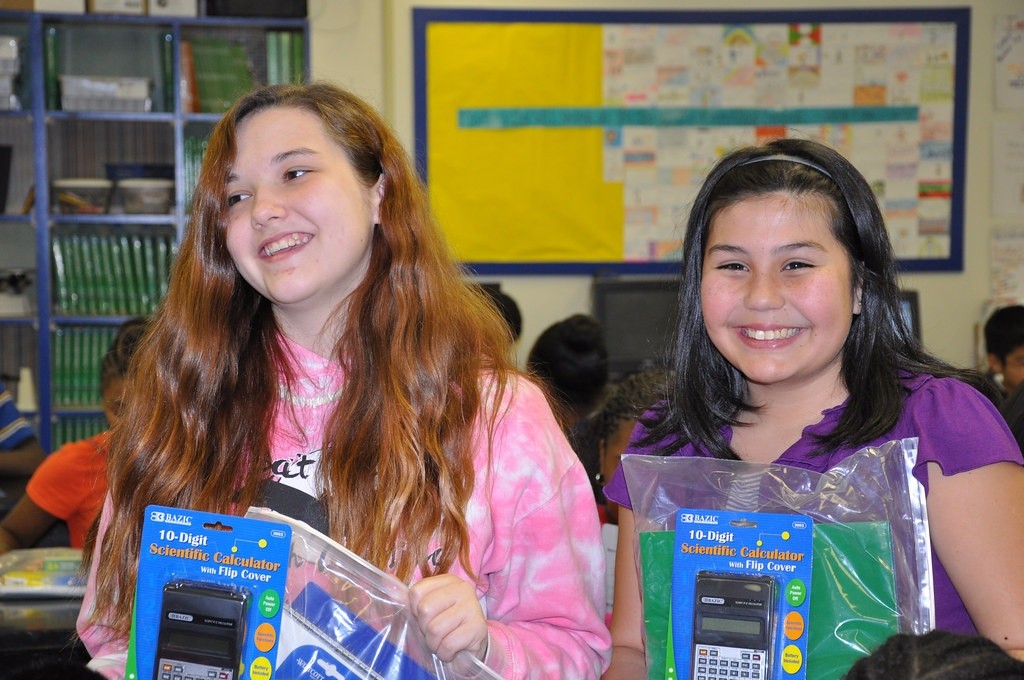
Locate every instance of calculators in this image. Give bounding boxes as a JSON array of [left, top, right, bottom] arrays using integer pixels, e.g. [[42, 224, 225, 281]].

[[691, 572, 774, 680], [152, 577, 248, 680]]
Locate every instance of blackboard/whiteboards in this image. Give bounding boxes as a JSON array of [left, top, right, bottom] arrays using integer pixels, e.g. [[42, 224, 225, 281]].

[[412, 7, 973, 278]]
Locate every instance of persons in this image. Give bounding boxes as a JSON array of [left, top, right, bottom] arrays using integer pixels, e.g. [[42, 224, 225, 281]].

[[76, 82, 613, 680], [492, 138, 1024, 680], [1, 318, 148, 553]]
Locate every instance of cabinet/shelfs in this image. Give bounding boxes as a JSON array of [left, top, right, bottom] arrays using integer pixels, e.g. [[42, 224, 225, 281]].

[[0, 9, 312, 453]]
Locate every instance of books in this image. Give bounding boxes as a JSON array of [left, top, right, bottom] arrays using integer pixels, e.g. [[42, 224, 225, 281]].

[[41, 25, 301, 447]]
